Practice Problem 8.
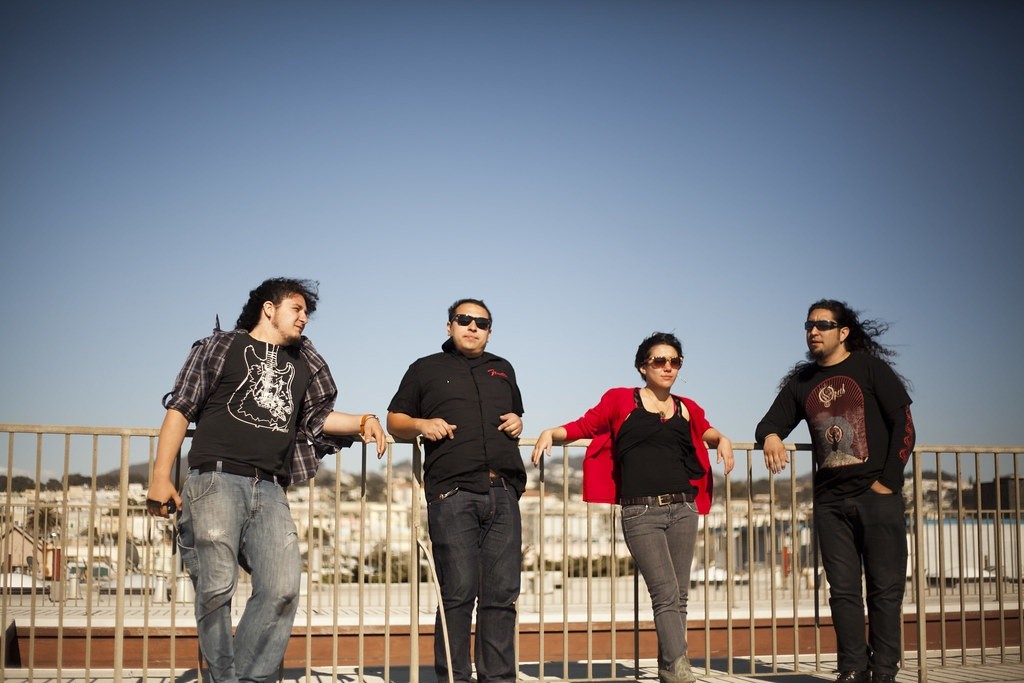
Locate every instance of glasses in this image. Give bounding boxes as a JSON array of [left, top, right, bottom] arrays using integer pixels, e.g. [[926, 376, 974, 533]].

[[450, 314, 492, 329], [641, 356, 683, 370], [805, 320, 844, 332]]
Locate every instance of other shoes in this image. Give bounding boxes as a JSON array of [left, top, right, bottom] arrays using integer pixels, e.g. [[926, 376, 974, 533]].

[[833, 671, 870, 683], [872, 671, 895, 683]]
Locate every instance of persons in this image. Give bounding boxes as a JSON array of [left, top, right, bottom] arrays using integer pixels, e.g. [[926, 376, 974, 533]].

[[146, 278, 388, 683], [754, 296, 917, 683], [386, 299, 526, 683], [530, 333, 736, 683]]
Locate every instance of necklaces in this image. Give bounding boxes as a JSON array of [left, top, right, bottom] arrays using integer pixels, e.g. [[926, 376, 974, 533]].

[[645, 388, 671, 418]]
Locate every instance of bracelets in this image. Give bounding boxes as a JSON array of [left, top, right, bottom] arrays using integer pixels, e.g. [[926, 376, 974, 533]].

[[360, 414, 379, 436], [764, 434, 778, 438]]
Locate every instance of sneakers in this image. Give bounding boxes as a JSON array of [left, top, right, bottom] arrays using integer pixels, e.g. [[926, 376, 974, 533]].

[[658, 655, 696, 683]]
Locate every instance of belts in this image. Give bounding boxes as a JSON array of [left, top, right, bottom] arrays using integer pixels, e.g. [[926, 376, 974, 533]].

[[190, 460, 291, 487], [621, 493, 694, 506], [488, 476, 508, 487]]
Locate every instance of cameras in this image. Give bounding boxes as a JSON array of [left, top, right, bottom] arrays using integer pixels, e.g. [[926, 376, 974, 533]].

[[167, 498, 176, 513]]
